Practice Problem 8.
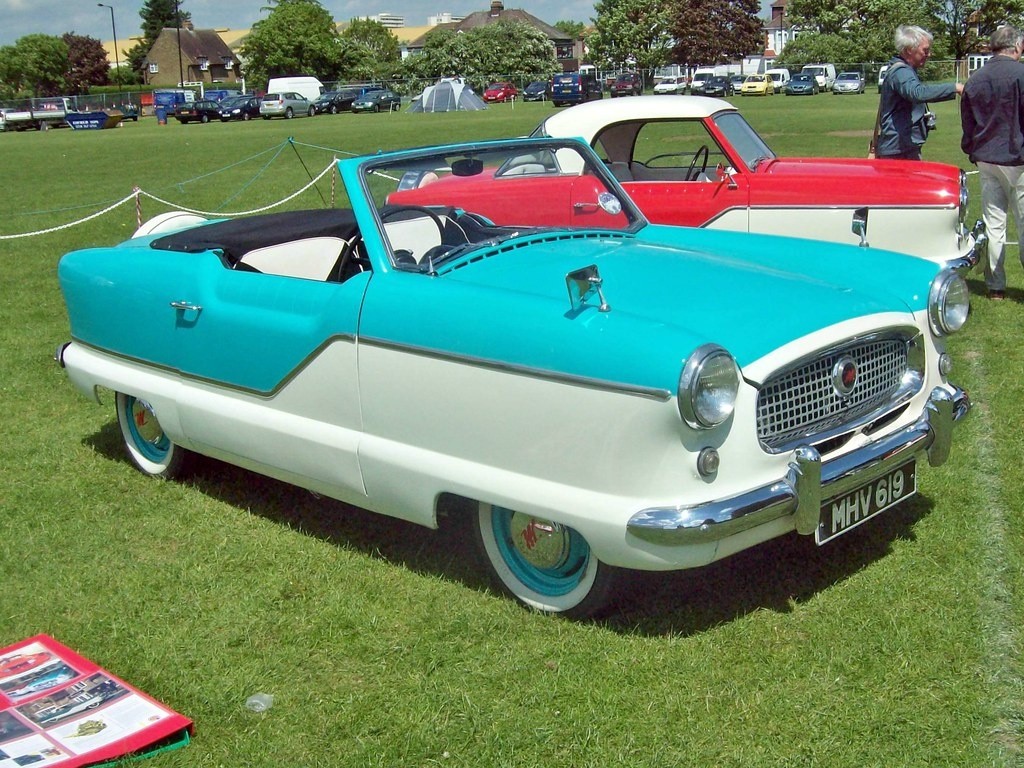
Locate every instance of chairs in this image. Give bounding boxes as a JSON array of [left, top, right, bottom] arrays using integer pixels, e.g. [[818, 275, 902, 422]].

[[755, 78, 759, 82], [618, 78, 625, 82], [229, 204, 477, 283], [796, 77, 801, 81]]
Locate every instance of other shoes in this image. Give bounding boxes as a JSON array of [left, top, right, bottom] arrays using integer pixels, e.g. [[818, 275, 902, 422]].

[[988, 289, 1004, 301]]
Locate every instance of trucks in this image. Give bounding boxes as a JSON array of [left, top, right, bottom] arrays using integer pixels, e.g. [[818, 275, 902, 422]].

[[268, 76, 326, 102], [691, 65, 742, 96], [5, 97, 80, 132]]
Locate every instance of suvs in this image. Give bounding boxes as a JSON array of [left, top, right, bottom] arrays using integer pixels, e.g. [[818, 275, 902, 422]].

[[609, 74, 643, 98]]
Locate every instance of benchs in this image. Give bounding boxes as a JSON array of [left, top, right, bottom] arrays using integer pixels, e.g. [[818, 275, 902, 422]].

[[586, 162, 635, 183]]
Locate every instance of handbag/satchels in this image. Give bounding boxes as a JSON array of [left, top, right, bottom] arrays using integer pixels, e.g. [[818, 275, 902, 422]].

[[867, 134, 880, 159]]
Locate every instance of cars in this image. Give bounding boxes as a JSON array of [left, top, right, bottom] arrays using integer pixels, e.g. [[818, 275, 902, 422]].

[[0, 108, 20, 131], [383, 95, 989, 277], [175, 99, 224, 124], [57, 134, 975, 622], [740, 74, 774, 96], [350, 90, 402, 114], [729, 73, 747, 94], [785, 73, 820, 96], [314, 90, 356, 115], [705, 75, 736, 97], [831, 72, 866, 95], [483, 82, 519, 104], [259, 92, 316, 120], [219, 94, 263, 122], [522, 82, 551, 101]]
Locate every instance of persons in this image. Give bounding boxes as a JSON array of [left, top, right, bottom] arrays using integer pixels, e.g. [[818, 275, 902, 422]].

[[960, 26, 1024, 301], [874, 24, 964, 160]]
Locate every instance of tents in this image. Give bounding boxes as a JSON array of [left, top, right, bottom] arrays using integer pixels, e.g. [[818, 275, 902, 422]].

[[404, 81, 489, 114]]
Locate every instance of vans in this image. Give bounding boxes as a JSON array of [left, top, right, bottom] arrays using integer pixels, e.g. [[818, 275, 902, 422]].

[[653, 75, 686, 95], [551, 74, 603, 108], [878, 65, 889, 95], [801, 64, 837, 92], [763, 69, 791, 94]]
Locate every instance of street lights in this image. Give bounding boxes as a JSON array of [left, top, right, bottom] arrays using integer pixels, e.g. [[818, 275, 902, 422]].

[[98, 4, 124, 100]]
